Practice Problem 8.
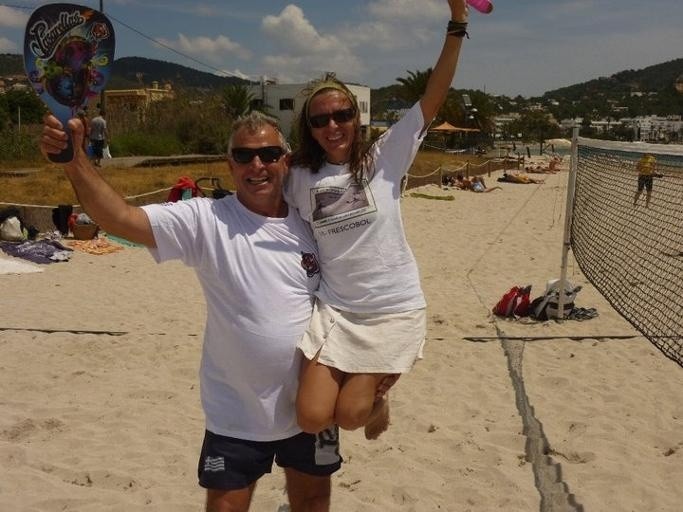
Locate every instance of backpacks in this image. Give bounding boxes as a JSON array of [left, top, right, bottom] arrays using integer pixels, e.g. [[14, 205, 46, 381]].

[[493, 285, 532, 320]]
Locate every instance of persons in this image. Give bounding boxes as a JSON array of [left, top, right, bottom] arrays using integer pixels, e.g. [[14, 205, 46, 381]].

[[501, 156, 562, 185], [36, 106, 403, 511], [87, 109, 112, 169], [456, 173, 504, 193], [446, 176, 488, 190], [276, 0, 475, 443], [75, 110, 90, 149], [633, 154, 664, 207]]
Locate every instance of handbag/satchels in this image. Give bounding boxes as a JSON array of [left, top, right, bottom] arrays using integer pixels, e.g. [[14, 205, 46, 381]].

[[535, 278, 577, 319], [88, 143, 93, 156]]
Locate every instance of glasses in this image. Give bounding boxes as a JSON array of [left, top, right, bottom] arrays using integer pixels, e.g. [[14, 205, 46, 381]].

[[231, 145, 286, 165], [307, 107, 357, 129]]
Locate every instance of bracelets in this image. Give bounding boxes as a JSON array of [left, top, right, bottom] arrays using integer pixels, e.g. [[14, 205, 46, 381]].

[[444, 21, 468, 39]]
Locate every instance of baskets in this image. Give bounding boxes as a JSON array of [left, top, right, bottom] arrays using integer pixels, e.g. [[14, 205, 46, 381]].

[[71, 223, 99, 240]]
